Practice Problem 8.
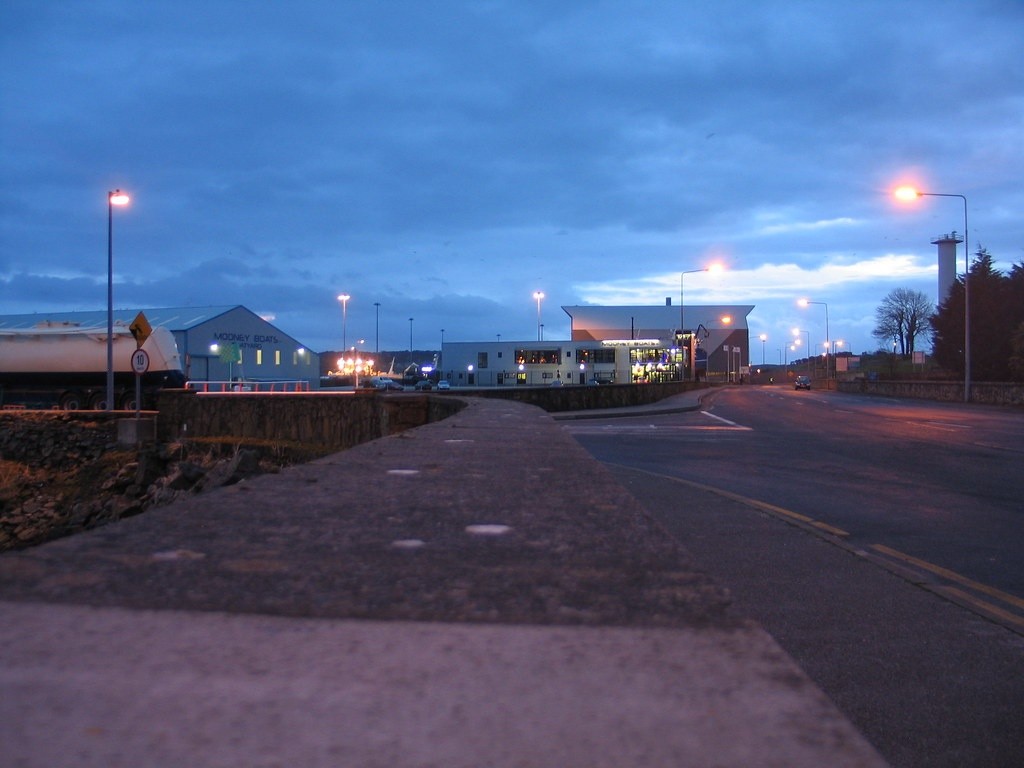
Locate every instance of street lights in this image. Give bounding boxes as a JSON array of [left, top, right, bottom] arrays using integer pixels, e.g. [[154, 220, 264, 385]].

[[106, 189, 131, 411], [705, 316, 730, 383], [680, 264, 725, 381], [799, 299, 831, 390], [832, 340, 843, 379], [793, 328, 811, 377], [892, 186, 972, 403], [784, 340, 800, 385], [540, 324, 544, 341], [532, 291, 544, 341], [373, 302, 382, 376], [336, 293, 351, 377], [814, 343, 829, 387], [496, 333, 502, 342], [439, 328, 446, 349], [407, 317, 414, 364]]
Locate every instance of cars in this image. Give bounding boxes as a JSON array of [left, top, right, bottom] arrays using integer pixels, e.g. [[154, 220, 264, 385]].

[[360, 374, 405, 391], [437, 380, 450, 390], [415, 381, 433, 391], [549, 380, 564, 387]]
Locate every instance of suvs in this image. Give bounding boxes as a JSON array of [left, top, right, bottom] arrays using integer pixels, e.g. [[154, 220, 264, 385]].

[[794, 376, 812, 390]]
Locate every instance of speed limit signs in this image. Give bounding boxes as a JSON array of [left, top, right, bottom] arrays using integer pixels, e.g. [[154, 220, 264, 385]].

[[130, 348, 149, 375]]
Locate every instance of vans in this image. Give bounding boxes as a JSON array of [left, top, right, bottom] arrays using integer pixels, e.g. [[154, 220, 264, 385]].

[[587, 378, 615, 385]]
